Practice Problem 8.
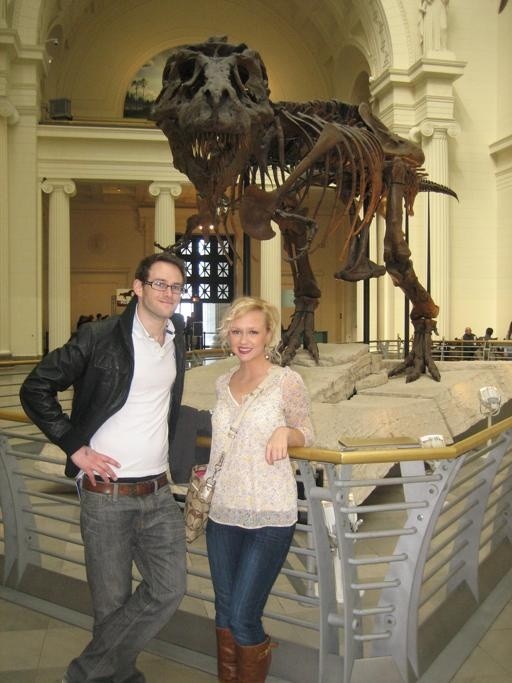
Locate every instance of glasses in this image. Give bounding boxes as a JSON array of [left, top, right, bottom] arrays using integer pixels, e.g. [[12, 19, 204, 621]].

[[142, 279, 186, 295]]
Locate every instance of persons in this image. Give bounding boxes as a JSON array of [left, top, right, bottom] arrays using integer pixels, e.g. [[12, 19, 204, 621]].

[[21, 253, 190, 683], [461, 321, 512, 359], [205, 298, 317, 683]]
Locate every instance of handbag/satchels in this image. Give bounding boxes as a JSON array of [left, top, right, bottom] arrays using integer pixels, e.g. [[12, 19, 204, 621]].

[[182, 461, 217, 544]]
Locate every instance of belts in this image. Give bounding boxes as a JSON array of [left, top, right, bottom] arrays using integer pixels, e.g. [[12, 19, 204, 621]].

[[80, 472, 172, 499]]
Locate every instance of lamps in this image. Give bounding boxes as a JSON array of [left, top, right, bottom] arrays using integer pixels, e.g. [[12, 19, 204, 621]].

[[480, 385, 502, 446]]
